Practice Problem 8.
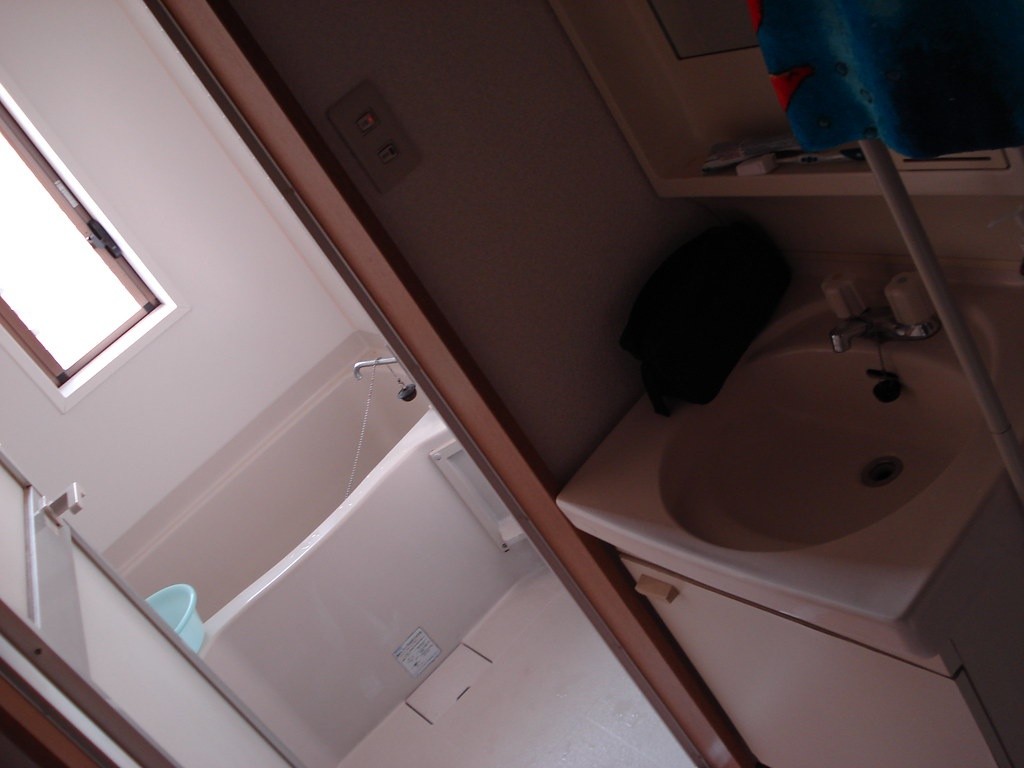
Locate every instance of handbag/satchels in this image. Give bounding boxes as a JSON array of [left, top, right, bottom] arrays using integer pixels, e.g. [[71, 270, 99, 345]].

[[619, 212, 794, 417]]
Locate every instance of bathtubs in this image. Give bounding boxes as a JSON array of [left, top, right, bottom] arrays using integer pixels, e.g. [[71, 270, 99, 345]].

[[97, 330, 546, 767]]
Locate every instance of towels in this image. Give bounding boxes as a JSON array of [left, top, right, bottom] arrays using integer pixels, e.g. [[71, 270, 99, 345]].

[[744, 0, 977, 160]]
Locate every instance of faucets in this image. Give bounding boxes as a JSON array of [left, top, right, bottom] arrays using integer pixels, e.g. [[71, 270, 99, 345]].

[[829, 311, 886, 352]]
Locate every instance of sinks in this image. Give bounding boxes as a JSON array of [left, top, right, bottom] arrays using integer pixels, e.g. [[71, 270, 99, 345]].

[[656, 347, 986, 553]]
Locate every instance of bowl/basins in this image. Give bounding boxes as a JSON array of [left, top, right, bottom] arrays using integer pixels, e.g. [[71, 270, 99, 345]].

[[144, 584, 204, 653]]
